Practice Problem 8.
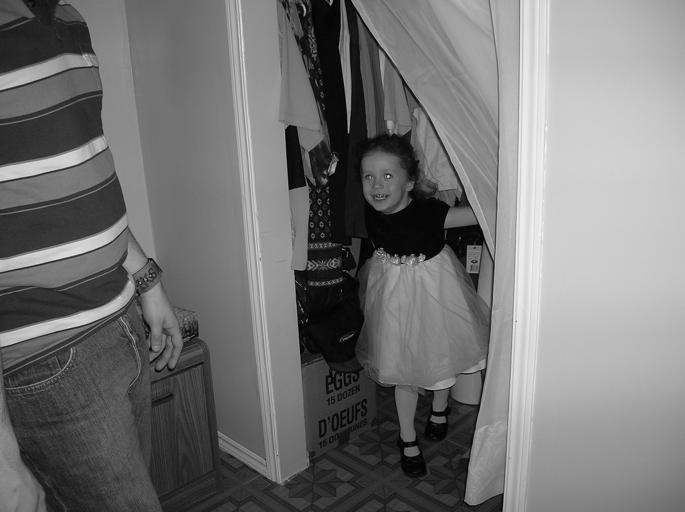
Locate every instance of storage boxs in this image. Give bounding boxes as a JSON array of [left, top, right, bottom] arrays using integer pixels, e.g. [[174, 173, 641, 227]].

[[298, 341, 380, 464]]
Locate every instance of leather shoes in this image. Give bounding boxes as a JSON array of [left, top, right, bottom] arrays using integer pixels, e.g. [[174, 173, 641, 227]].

[[424, 400, 452, 441], [397, 429, 427, 479]]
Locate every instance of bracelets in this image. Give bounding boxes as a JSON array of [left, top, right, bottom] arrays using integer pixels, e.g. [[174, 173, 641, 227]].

[[132, 257, 164, 296]]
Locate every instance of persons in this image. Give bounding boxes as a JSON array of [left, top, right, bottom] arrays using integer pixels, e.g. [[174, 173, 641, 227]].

[[348, 133, 491, 480], [0, 1, 184, 512]]
